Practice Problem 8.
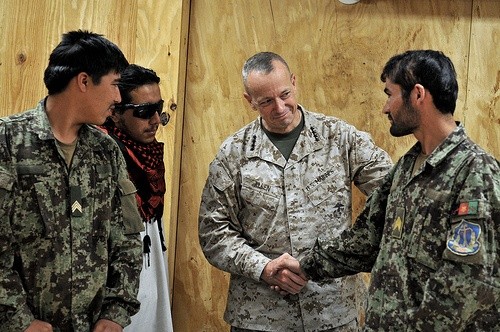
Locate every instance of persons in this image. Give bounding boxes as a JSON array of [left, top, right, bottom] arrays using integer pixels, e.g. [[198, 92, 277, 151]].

[[0, 29, 146, 332], [198, 51, 394, 332], [94, 63, 163, 332], [270, 49, 500, 332]]
